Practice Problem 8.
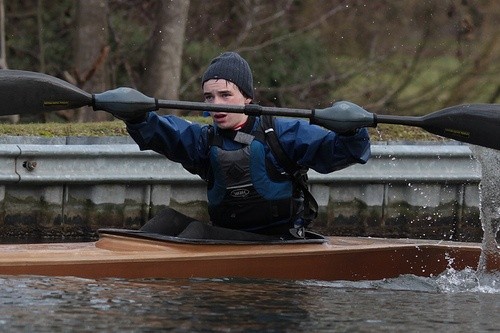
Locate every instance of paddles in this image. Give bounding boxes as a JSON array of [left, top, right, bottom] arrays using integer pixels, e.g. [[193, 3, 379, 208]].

[[0, 68, 500, 151]]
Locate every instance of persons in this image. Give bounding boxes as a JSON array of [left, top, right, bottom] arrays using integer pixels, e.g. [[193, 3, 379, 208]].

[[96, 51, 372, 240]]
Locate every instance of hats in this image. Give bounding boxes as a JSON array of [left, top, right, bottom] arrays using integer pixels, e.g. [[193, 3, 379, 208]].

[[202, 51, 254, 100]]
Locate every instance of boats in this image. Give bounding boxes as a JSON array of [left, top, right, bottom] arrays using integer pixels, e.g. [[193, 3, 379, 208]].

[[0, 234, 499, 296]]
